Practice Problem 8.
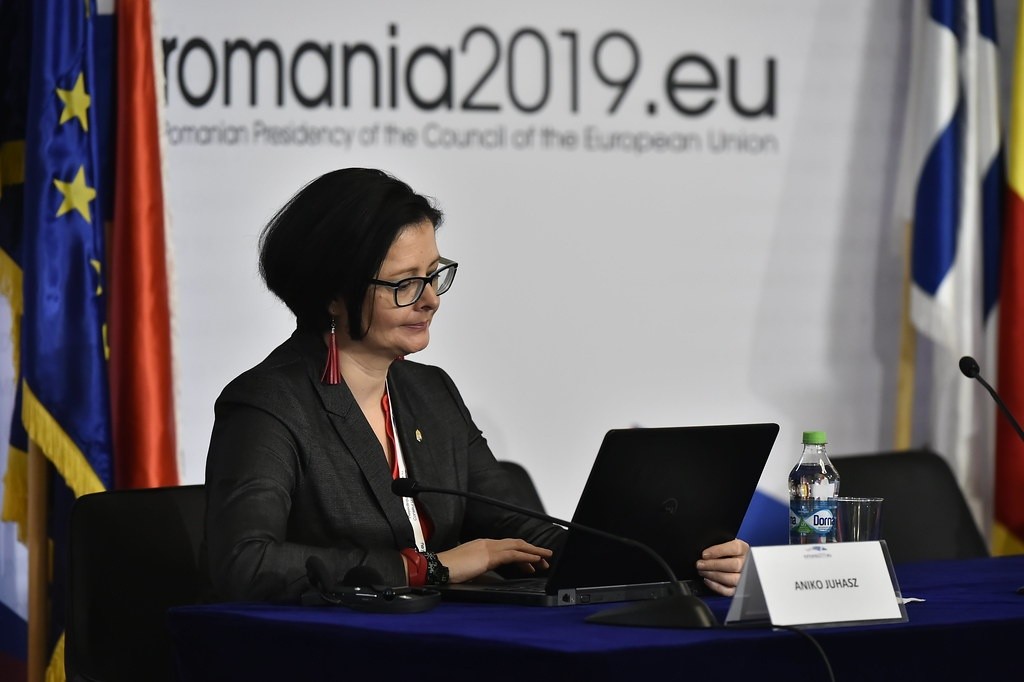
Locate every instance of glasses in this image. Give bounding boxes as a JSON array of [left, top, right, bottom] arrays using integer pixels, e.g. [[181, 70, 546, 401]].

[[372, 257, 458, 307]]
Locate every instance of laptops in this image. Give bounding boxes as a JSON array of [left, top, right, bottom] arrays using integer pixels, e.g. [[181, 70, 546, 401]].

[[441, 423, 780, 607]]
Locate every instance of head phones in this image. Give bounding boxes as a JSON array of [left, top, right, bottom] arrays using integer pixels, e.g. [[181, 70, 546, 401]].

[[306, 554, 441, 613]]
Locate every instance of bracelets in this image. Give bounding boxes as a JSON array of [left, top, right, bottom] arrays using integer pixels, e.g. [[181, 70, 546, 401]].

[[418, 549, 451, 585]]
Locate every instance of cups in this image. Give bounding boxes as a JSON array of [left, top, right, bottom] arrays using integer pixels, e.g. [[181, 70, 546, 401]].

[[833, 494, 884, 544]]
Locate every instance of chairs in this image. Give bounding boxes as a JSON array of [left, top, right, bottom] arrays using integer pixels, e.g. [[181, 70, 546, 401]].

[[801, 446, 990, 562], [65, 484, 233, 607]]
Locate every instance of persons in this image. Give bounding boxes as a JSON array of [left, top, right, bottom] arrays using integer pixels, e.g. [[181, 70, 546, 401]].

[[193, 167, 754, 618]]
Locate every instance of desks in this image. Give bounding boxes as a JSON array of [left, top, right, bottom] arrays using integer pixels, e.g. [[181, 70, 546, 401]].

[[198, 551, 1023, 680]]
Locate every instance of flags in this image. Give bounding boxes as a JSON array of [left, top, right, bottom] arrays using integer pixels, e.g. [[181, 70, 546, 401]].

[[886, 1, 1024, 559], [1, 0, 183, 680]]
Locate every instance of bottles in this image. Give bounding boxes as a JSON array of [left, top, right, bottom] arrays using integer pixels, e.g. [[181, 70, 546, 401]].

[[785, 432, 840, 544]]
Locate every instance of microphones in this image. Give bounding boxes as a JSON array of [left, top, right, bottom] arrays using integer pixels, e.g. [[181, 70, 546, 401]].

[[959, 356, 1023, 441], [391, 478, 719, 629]]
